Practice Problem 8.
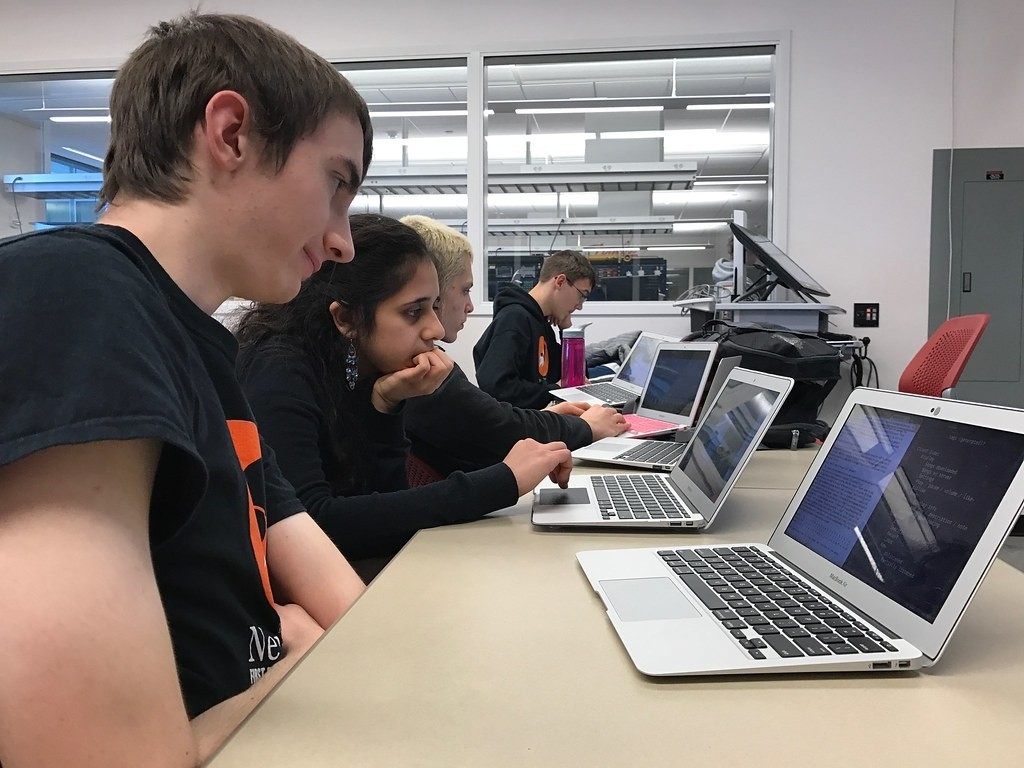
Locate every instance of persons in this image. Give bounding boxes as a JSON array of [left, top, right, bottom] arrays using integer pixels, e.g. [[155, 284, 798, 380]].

[[0, 14, 375, 768], [472, 250, 590, 410], [236, 215, 573, 585], [400, 215, 632, 488]]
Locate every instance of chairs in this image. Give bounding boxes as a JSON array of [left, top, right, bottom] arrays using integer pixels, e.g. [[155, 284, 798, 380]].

[[898, 313, 991, 399]]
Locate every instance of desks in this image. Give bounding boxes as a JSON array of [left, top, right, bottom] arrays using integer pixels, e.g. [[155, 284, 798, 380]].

[[198, 487, 1024, 768], [545, 400, 821, 488]]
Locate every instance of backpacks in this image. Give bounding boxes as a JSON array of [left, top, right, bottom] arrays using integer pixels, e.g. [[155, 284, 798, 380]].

[[679, 320, 841, 451]]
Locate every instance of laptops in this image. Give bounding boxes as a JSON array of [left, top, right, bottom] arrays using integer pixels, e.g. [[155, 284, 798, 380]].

[[532, 366, 794, 531], [549, 332, 683, 406], [572, 355, 742, 471], [615, 342, 718, 438], [575, 386, 1024, 676]]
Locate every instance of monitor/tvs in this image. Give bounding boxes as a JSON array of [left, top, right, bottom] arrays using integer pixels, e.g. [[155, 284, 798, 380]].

[[729, 222, 831, 297]]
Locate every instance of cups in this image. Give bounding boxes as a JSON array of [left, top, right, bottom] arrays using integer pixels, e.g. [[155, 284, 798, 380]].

[[561, 328, 585, 388]]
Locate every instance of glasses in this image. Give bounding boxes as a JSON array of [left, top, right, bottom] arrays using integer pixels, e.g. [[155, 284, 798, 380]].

[[555, 275, 588, 302]]
